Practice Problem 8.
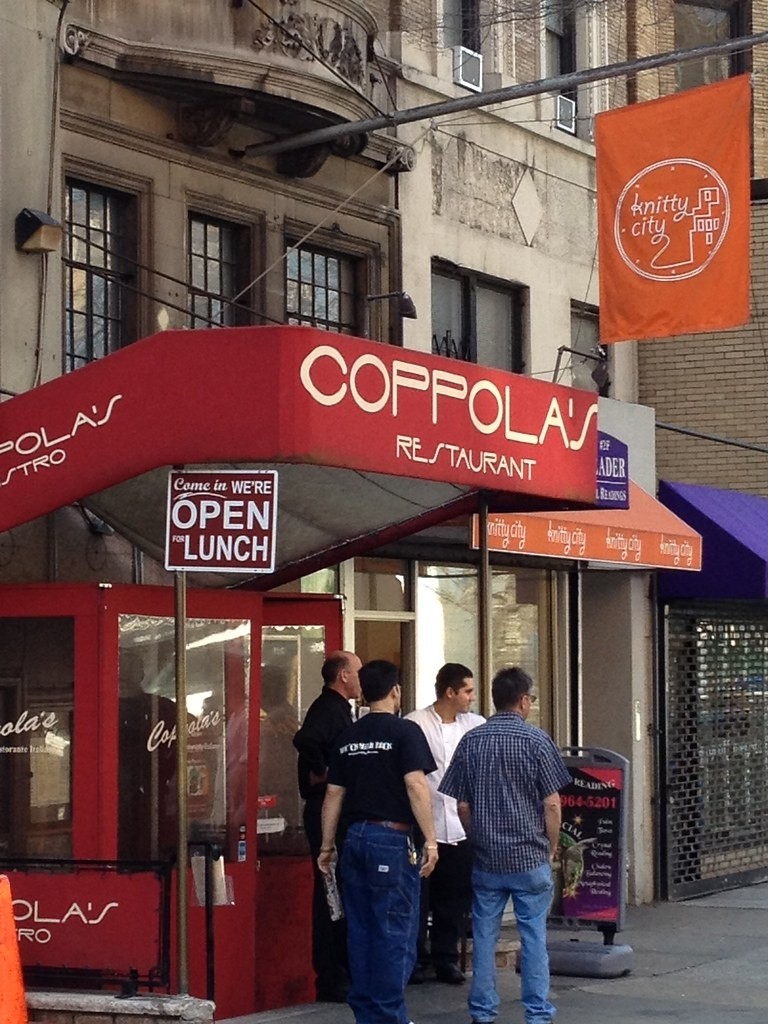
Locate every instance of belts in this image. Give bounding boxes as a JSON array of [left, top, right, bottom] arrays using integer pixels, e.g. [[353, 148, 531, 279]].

[[369, 819, 413, 834]]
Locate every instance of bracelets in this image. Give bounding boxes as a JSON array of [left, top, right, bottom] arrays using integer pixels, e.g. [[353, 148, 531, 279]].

[[321, 847, 334, 852], [424, 844, 437, 849]]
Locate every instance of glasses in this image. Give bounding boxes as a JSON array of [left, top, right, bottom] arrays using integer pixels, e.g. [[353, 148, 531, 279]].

[[521, 693, 536, 703]]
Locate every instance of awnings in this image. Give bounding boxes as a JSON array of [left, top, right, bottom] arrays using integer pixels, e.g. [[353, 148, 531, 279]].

[[656, 481, 768, 600], [460, 478, 702, 571]]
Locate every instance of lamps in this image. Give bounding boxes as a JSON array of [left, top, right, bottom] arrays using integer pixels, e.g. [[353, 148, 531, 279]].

[[16, 207, 61, 252], [369, 291, 418, 320]]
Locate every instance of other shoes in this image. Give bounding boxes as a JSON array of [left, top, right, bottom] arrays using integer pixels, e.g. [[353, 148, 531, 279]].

[[435, 962, 466, 983], [409, 966, 424, 983]]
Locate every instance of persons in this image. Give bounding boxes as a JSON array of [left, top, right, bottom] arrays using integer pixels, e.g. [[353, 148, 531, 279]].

[[293, 650, 362, 1003], [211, 662, 267, 829], [437, 665, 573, 1024], [403, 661, 487, 986], [116, 651, 176, 864], [255, 669, 303, 832], [312, 658, 439, 1024]]
